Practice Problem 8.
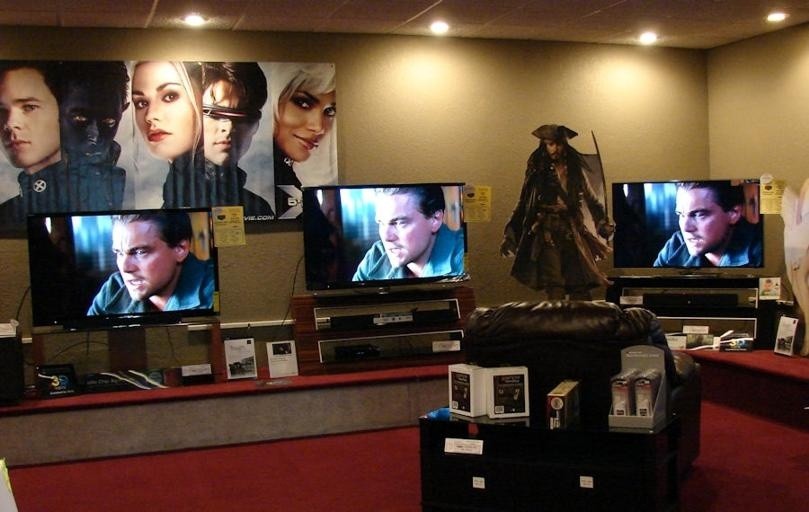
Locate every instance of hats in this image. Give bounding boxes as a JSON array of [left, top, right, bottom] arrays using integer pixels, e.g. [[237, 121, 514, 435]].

[[532, 124, 578, 139]]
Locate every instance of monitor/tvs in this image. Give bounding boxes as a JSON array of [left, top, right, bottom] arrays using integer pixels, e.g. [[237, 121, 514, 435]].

[[302, 182, 468, 297], [612, 177, 764, 279], [25, 207, 221, 333]]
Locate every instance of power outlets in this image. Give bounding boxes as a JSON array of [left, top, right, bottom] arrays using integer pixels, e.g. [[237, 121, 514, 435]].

[[205, 325, 257, 345]]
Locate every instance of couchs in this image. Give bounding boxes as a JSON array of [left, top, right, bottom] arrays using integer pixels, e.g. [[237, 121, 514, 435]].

[[623, 306, 701, 480], [418, 301, 680, 512]]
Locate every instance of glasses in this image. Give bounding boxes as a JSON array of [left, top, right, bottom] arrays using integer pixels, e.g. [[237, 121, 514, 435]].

[[202, 106, 265, 121]]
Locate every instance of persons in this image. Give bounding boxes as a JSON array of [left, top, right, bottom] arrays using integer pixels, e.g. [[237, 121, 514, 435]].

[[192, 63, 274, 216], [2, 64, 77, 220], [133, 62, 201, 208], [60, 59, 130, 213], [269, 64, 335, 218], [351, 186, 462, 279], [85, 212, 214, 310], [501, 125, 616, 299], [655, 182, 765, 267]]
